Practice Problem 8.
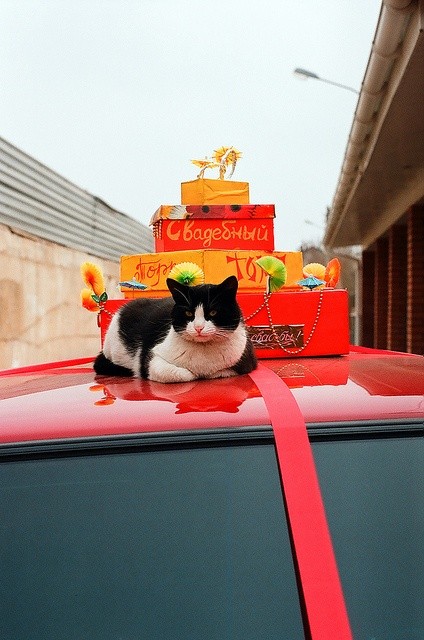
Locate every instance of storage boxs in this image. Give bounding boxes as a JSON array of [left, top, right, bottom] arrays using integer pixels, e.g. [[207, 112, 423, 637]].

[[119, 253, 303, 292], [100, 288, 349, 359], [181, 179, 249, 205], [148, 205, 275, 253]]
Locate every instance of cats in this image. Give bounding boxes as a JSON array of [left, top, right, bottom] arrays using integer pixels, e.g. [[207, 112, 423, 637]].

[[93, 275, 257, 384], [94, 375, 248, 414]]
